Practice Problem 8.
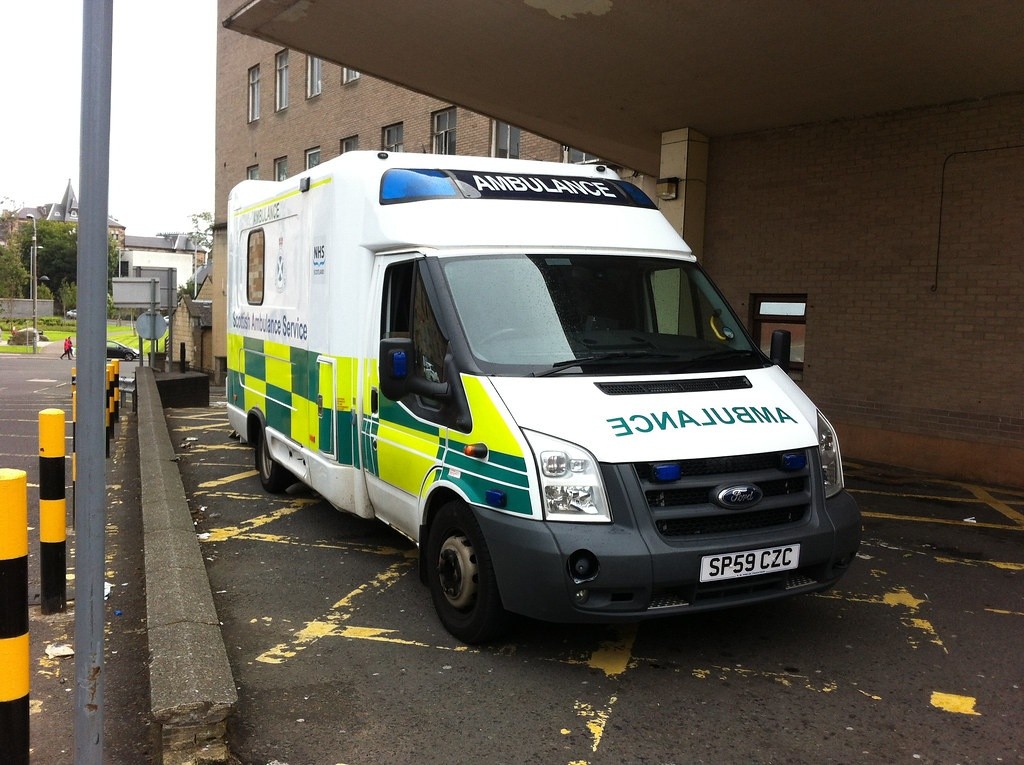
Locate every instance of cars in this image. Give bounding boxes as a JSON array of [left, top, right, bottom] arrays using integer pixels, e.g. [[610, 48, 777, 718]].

[[105, 340, 141, 361]]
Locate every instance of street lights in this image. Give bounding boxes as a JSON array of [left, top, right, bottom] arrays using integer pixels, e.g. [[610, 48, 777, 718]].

[[30, 245, 43, 302], [26, 214, 38, 354]]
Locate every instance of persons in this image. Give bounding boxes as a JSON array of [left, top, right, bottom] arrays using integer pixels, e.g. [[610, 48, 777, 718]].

[[60, 337, 74, 360]]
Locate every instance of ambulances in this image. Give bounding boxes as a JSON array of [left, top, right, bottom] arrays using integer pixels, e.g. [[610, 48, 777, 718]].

[[224, 148, 863, 643]]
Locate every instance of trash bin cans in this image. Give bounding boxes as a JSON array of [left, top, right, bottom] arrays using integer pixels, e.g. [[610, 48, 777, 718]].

[[37, 329, 43, 336], [148, 352, 167, 373]]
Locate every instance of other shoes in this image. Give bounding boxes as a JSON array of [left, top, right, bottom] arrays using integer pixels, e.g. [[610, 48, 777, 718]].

[[60, 357, 62, 359], [68, 358, 72, 360]]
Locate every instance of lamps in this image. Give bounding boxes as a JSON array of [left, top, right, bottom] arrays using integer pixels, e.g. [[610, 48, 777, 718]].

[[652, 177, 679, 201]]
